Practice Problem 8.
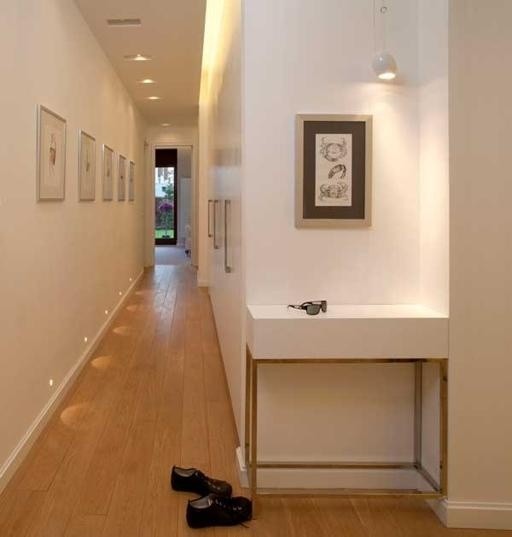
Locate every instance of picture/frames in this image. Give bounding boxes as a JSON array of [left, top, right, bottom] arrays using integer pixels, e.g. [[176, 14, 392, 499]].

[[208, 198, 233, 272], [36, 102, 135, 201], [297, 115, 374, 230]]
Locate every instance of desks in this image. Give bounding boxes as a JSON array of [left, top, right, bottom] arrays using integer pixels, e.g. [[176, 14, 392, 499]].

[[245, 303, 448, 500]]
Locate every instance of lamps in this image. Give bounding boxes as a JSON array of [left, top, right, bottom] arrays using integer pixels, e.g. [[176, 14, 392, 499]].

[[374, 0, 397, 79]]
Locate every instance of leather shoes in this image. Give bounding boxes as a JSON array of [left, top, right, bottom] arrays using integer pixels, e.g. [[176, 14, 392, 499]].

[[185, 494, 252, 529], [170, 464, 232, 498]]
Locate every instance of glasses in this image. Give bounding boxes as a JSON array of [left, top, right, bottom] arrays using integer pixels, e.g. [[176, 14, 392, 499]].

[[286, 300, 327, 315]]
